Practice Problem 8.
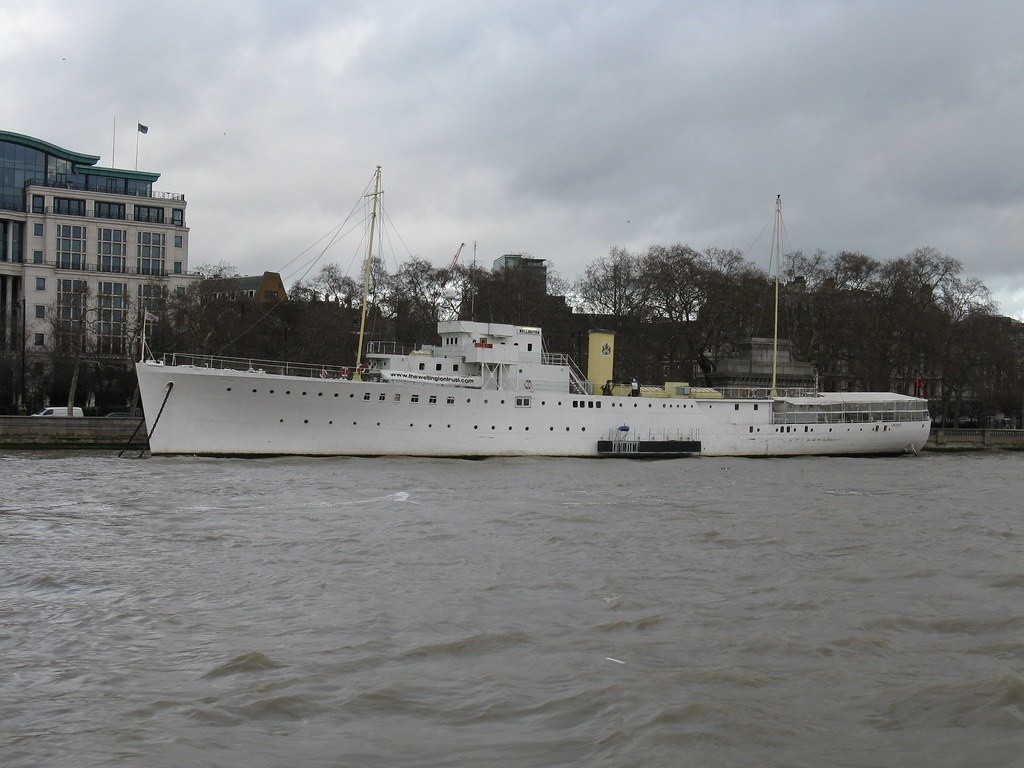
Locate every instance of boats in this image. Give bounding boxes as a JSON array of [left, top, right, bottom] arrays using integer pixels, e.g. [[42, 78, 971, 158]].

[[136, 165, 932, 457]]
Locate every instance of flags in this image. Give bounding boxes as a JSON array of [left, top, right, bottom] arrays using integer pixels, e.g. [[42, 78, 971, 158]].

[[917, 373, 923, 388], [138, 123, 149, 134]]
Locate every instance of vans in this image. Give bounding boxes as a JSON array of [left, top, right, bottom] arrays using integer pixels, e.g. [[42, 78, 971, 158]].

[[32, 406, 84, 417]]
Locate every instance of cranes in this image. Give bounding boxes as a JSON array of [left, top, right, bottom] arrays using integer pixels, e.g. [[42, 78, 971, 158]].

[[431, 242, 465, 304]]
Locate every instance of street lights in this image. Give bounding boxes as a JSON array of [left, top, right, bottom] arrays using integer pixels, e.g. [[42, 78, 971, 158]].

[[17, 297, 26, 406], [352, 318, 357, 361]]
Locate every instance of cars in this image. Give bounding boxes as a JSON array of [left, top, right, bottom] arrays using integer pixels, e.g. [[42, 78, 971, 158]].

[[105, 412, 133, 417]]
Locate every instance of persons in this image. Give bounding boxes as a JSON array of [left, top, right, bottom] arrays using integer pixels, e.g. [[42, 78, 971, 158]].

[[1001, 416, 1007, 428], [923, 412, 929, 420], [628, 377, 640, 396], [841, 413, 894, 423], [992, 418, 999, 428], [923, 387, 929, 399]]
